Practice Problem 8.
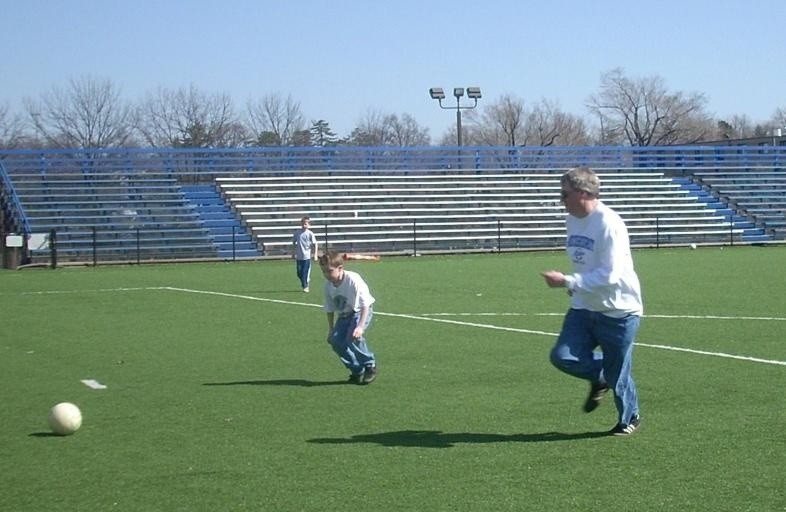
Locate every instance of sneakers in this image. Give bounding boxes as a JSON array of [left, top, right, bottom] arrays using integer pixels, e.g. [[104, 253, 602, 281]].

[[348, 374, 363, 383], [609, 414, 641, 436], [583, 378, 611, 414], [363, 363, 377, 383], [303, 286, 311, 293]]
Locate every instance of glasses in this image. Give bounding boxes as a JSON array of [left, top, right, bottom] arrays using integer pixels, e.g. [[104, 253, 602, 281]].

[[559, 188, 581, 201]]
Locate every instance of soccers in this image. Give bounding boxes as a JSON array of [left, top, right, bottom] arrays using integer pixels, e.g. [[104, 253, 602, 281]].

[[47, 401, 82, 435]]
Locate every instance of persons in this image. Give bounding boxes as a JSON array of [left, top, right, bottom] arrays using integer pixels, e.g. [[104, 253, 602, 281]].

[[539, 167, 644, 437], [318, 250, 379, 384], [290, 216, 318, 294]]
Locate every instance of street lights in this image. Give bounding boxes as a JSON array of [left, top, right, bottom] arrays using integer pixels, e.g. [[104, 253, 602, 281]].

[[430, 87, 482, 174]]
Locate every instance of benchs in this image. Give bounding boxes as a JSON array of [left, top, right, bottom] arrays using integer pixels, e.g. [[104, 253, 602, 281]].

[[0, 165, 223, 260], [220, 168, 572, 257], [589, 166, 786, 249]]
